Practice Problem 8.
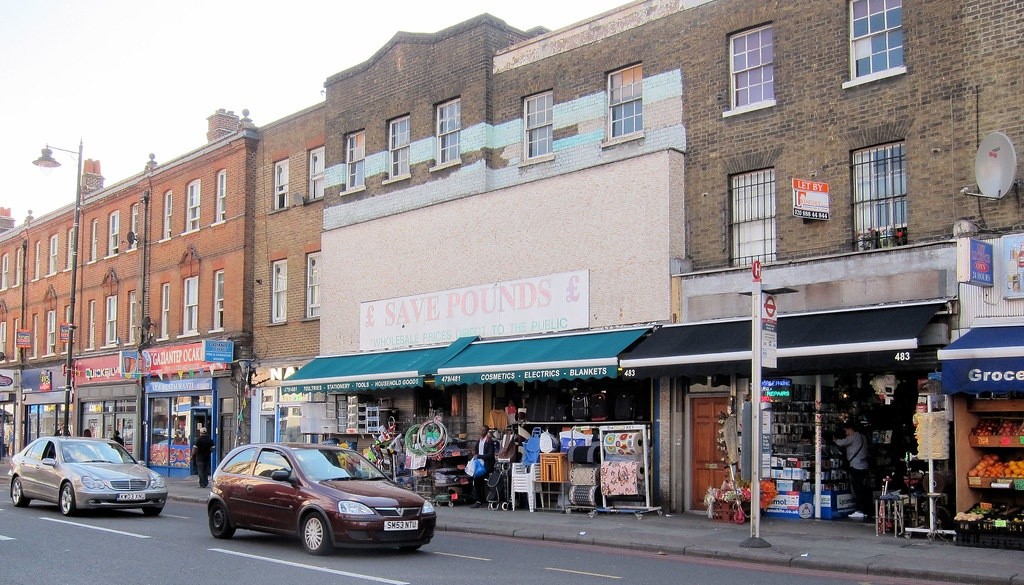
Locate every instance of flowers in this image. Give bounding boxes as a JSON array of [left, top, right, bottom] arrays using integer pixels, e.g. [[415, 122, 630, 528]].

[[855, 228, 907, 249]]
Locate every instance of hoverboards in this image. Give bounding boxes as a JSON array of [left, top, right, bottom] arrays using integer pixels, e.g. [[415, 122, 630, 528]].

[[723, 463, 750, 524]]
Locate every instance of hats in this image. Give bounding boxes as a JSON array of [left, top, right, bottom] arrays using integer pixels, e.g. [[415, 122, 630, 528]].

[[200, 427, 207, 433]]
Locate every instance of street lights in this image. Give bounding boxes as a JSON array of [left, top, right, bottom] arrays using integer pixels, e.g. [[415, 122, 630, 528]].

[[32, 140, 83, 431]]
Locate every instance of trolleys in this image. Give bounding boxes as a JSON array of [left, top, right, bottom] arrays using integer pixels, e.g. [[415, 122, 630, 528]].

[[484, 471, 508, 511]]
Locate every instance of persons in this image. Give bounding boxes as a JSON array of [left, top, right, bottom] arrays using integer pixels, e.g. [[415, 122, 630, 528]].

[[111, 431, 124, 456], [191, 427, 215, 488], [54, 423, 71, 436], [84, 429, 91, 437], [471, 425, 495, 508], [833, 424, 871, 517]]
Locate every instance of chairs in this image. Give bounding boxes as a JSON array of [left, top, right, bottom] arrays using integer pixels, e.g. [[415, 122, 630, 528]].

[[511, 463, 544, 513]]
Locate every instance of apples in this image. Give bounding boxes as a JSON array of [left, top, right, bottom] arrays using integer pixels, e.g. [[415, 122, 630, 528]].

[[969, 419, 1024, 436], [969, 454, 1006, 478]]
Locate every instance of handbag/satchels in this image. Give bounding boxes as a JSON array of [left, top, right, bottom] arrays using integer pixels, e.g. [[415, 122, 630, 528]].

[[465, 456, 486, 477], [841, 459, 849, 471]]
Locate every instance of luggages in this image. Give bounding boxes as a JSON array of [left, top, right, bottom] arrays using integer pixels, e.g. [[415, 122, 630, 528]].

[[521, 427, 541, 473], [496, 430, 518, 470], [486, 463, 511, 510], [525, 376, 650, 422]]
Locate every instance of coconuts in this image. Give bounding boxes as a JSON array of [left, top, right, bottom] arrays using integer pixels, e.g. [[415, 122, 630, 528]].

[[954, 511, 984, 521]]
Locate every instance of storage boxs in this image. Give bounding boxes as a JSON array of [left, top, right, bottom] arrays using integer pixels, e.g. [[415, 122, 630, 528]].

[[954, 503, 1024, 550], [299, 392, 347, 434]]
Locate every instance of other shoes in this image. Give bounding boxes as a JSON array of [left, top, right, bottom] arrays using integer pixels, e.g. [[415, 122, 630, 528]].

[[471, 502, 480, 509], [849, 511, 864, 518]]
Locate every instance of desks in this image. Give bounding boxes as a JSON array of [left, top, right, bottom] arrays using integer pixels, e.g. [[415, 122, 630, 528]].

[[875, 495, 909, 539]]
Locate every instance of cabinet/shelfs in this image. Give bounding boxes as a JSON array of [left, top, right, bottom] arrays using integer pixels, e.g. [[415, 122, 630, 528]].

[[966, 399, 1024, 491], [532, 481, 570, 514], [433, 439, 478, 507]]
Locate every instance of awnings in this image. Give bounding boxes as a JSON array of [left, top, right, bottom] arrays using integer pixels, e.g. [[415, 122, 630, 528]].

[[620, 303, 943, 380], [281, 348, 445, 394], [435, 329, 650, 386], [938, 325, 1024, 394]]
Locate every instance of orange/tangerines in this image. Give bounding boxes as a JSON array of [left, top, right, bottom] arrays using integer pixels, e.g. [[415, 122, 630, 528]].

[[1004, 460, 1024, 478]]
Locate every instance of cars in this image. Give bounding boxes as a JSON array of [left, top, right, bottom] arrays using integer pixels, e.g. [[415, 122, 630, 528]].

[[207, 442, 437, 555], [8, 436, 168, 518]]
